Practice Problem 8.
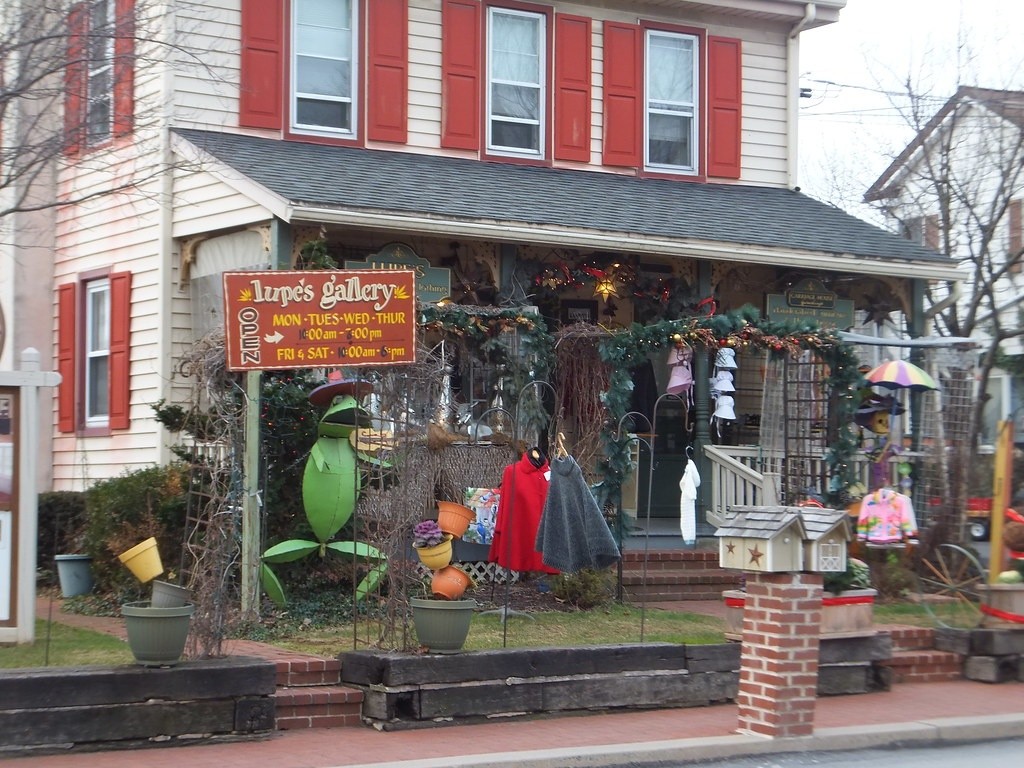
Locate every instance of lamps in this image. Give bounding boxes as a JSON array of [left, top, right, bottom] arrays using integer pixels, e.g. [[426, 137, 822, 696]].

[[592, 278, 619, 303]]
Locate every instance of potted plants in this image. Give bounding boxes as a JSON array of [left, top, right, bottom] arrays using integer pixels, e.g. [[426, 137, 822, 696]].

[[976, 568, 1024, 630], [722, 556, 879, 642], [412, 520, 454, 570], [431, 562, 478, 601]]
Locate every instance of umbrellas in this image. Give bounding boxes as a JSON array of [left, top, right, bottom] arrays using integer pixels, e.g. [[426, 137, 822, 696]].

[[862, 359, 939, 437]]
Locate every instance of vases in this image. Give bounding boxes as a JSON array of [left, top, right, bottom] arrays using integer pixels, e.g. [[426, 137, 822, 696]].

[[121, 601, 195, 666], [410, 595, 476, 655], [438, 501, 476, 541], [152, 580, 194, 609], [55, 554, 94, 598], [118, 536, 164, 583]]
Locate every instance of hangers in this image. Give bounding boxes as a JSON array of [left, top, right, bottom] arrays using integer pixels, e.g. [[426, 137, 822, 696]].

[[525, 432, 539, 459], [882, 477, 886, 499], [686, 446, 694, 459], [556, 433, 568, 460]]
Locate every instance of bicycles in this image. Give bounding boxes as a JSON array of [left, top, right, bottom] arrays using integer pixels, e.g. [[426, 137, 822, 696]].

[[873, 536, 987, 637]]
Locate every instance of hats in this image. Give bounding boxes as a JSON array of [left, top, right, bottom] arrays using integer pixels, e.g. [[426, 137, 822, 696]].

[[713, 347, 738, 377], [713, 372, 736, 393], [667, 340, 694, 372], [667, 365, 691, 413], [710, 395, 736, 438]]
